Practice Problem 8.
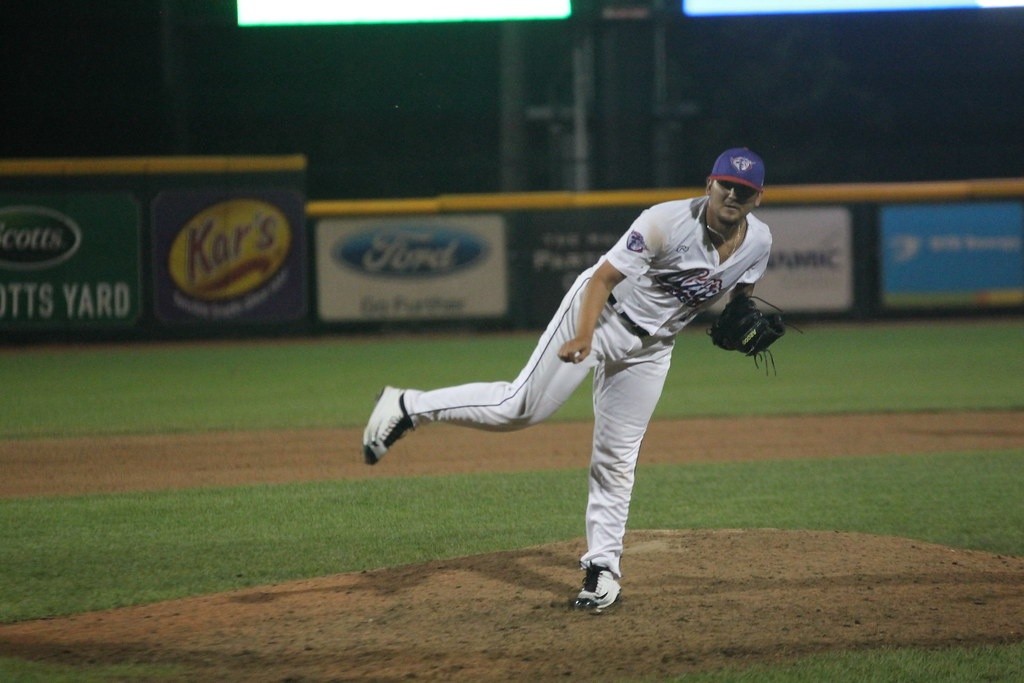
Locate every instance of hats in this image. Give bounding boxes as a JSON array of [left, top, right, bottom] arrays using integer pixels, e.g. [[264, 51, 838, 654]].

[[710, 148, 764, 194]]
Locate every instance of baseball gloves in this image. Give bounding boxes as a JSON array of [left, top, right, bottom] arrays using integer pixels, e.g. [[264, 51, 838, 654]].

[[705, 292, 806, 378]]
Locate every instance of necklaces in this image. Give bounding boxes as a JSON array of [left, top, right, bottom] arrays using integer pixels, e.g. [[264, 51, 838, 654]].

[[707, 222, 741, 256]]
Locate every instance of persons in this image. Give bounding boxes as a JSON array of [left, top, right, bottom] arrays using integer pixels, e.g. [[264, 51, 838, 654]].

[[363, 147, 787, 615]]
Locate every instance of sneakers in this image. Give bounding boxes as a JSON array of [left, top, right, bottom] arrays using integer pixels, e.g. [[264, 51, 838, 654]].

[[361, 385, 418, 465], [572, 559, 621, 612]]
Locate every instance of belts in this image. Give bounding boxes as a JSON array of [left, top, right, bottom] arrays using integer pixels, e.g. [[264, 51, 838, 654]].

[[608, 293, 649, 336]]
